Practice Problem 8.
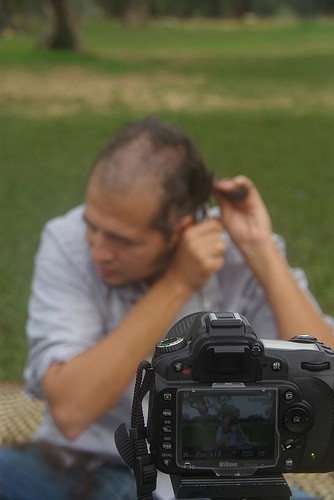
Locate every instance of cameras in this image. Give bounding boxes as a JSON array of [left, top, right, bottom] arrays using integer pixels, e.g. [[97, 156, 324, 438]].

[[144, 312, 334, 500]]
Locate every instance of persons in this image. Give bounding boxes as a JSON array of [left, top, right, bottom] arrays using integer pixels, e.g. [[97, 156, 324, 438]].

[[0, 116, 334, 500], [216, 416, 249, 455]]
